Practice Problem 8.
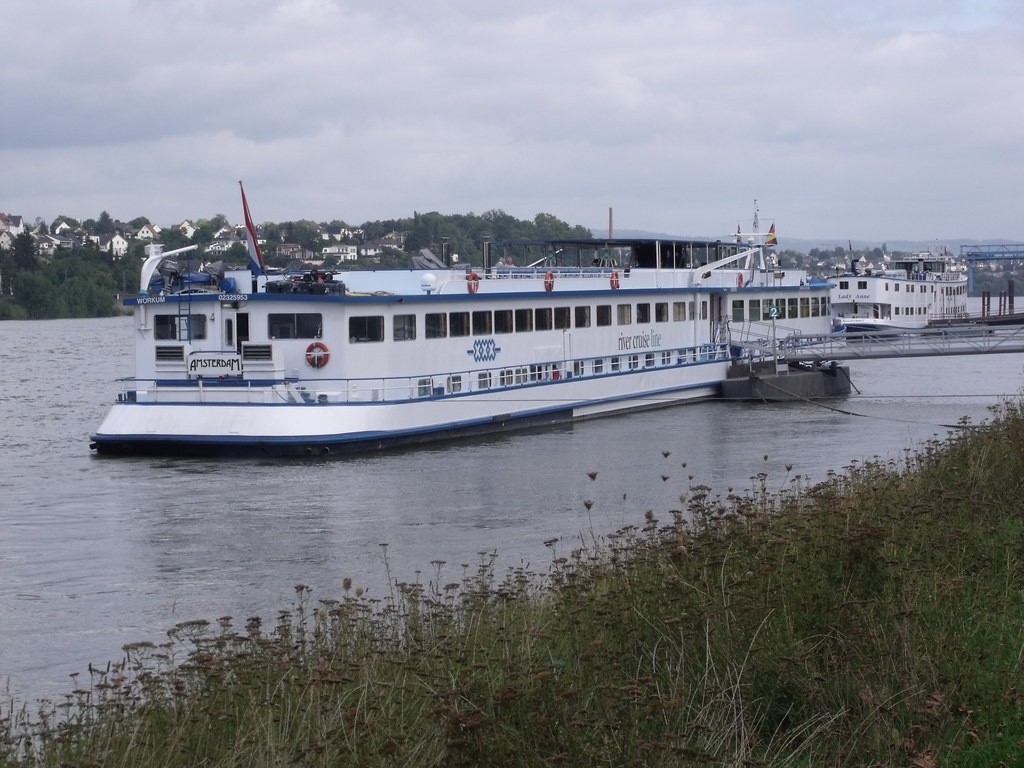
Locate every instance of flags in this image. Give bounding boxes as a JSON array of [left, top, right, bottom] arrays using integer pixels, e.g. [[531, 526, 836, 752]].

[[242, 193, 262, 280], [765, 224, 777, 247]]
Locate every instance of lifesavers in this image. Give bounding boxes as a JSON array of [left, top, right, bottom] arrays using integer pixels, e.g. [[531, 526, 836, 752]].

[[736, 273, 743, 287], [306, 342, 329, 367], [544, 271, 554, 291], [468, 273, 479, 293], [611, 272, 619, 289]]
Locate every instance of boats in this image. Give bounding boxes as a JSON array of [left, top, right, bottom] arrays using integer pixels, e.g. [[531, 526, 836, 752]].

[[822, 240, 969, 343], [89, 227, 848, 463]]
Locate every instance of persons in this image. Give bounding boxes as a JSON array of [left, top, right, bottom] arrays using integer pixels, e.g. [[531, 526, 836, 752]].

[[505, 257, 514, 267], [495, 258, 506, 267]]
